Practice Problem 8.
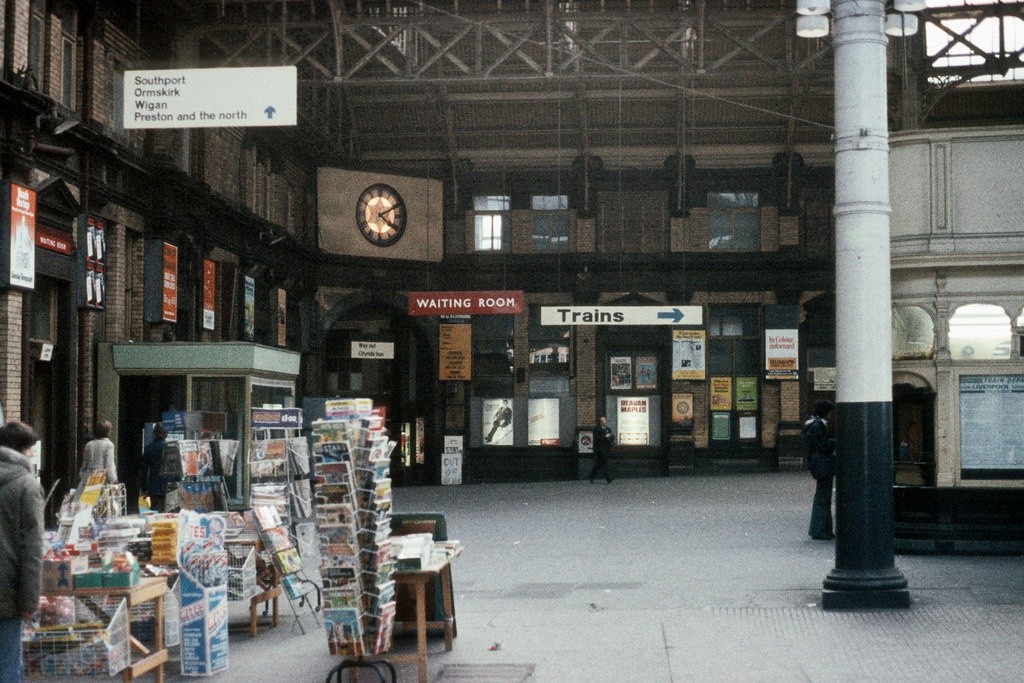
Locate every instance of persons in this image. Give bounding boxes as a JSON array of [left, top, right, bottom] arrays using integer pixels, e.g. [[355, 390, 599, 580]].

[[0, 421, 44, 683], [802, 399, 836, 540], [140, 423, 169, 513], [78, 419, 118, 511], [484, 400, 512, 443], [589, 417, 616, 484]]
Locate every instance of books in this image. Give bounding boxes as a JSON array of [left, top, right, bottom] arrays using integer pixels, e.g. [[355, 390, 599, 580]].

[[177, 400, 397, 656]]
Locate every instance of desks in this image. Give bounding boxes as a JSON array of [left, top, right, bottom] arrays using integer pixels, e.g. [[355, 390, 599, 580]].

[[348, 540, 464, 683], [40, 576, 168, 683], [224, 534, 282, 638]]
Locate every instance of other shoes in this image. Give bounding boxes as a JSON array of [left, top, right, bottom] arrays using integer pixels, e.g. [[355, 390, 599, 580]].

[[587, 476, 593, 484], [607, 477, 615, 483], [812, 532, 836, 540]]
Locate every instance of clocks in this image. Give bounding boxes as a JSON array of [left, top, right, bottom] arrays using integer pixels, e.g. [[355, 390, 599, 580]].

[[356, 183, 408, 247]]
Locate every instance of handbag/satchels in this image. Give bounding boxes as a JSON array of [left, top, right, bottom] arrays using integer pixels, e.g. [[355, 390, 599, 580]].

[[134, 455, 152, 485]]
